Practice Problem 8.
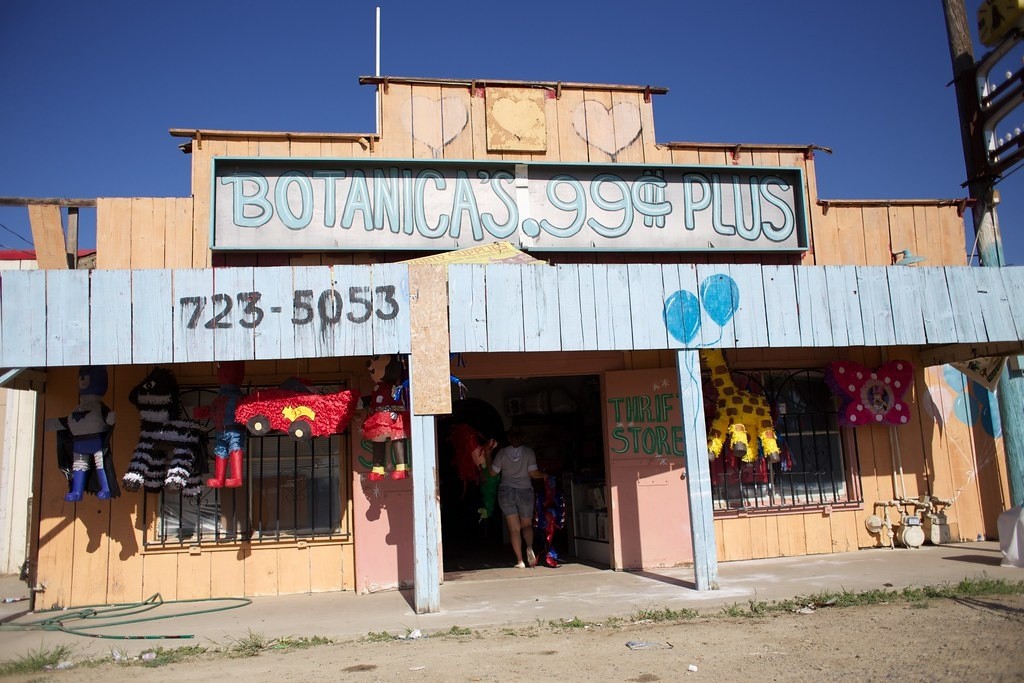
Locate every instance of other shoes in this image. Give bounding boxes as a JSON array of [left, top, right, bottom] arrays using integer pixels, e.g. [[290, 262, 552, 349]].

[[526, 548, 536, 566], [514, 563, 526, 568]]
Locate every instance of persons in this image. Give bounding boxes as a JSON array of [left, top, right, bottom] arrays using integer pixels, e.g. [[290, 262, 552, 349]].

[[484, 426, 547, 568]]
